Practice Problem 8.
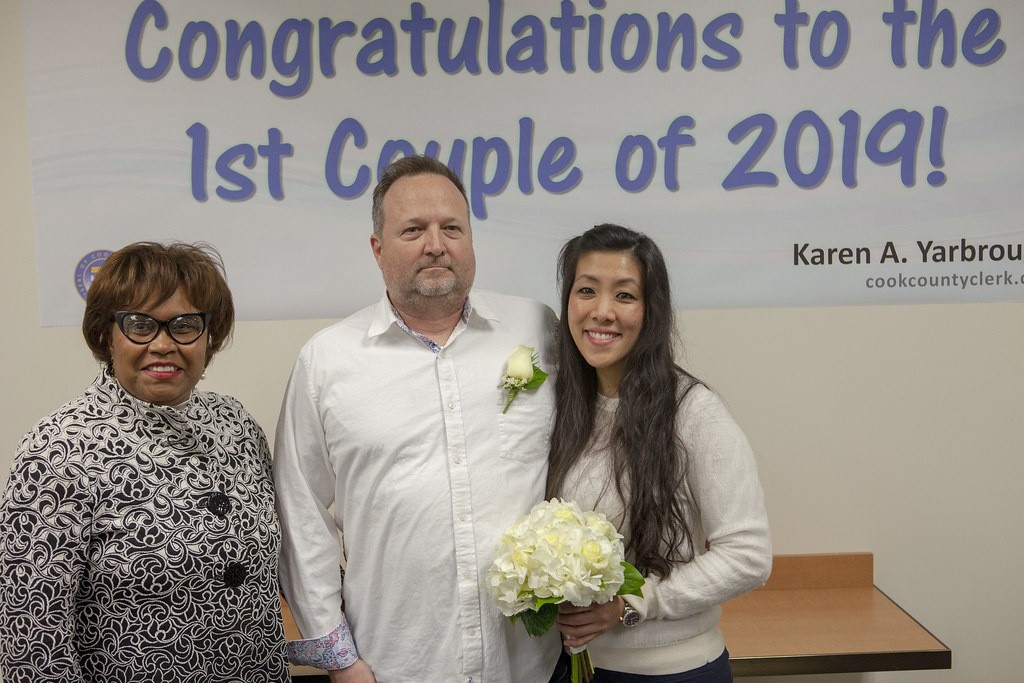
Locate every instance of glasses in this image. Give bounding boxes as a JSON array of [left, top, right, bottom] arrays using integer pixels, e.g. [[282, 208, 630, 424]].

[[113, 309, 207, 346]]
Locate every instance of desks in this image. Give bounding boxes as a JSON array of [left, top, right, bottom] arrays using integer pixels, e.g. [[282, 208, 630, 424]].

[[716, 550, 949, 676]]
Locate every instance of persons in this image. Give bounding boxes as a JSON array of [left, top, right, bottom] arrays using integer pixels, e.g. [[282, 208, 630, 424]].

[[2, 241, 293, 683], [543, 223, 773, 683], [268, 154, 573, 683]]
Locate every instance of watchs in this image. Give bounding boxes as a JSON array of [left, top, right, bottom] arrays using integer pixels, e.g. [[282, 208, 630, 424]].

[[619, 599, 640, 629]]
